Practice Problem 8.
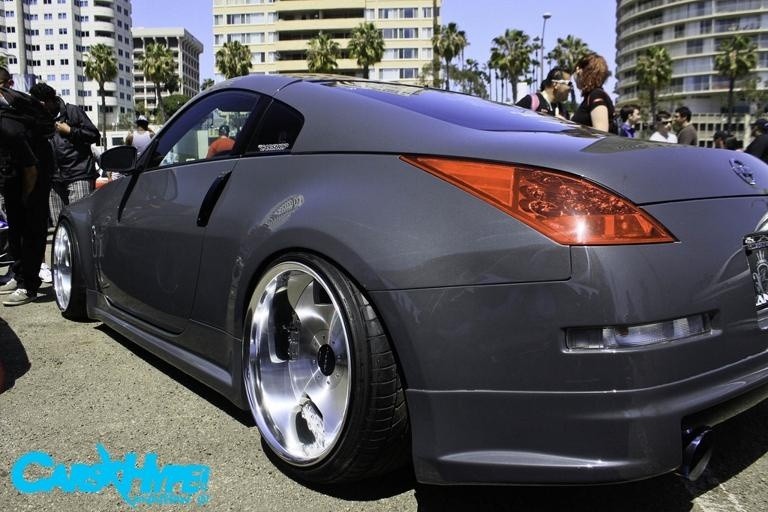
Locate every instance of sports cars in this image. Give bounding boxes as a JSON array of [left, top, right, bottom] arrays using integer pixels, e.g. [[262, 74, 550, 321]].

[[48, 70, 768, 490]]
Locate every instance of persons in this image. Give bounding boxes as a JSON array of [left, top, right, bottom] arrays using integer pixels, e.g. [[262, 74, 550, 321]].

[[618, 104, 642, 139], [38, 255, 53, 283], [744, 119, 768, 165], [126, 112, 156, 160], [206, 125, 236, 157], [0, 67, 56, 305], [712, 131, 731, 150], [647, 112, 677, 145], [27, 83, 101, 227], [569, 54, 614, 135], [516, 66, 572, 122], [673, 108, 698, 146]]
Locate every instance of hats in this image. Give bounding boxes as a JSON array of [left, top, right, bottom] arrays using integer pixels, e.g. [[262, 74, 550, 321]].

[[749, 118, 767, 127], [134, 115, 150, 122], [219, 125, 230, 132], [716, 131, 726, 144]]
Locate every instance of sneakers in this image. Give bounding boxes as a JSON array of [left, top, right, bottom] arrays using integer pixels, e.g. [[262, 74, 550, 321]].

[[2, 288, 38, 306], [38, 263, 53, 282], [0, 277, 17, 295]]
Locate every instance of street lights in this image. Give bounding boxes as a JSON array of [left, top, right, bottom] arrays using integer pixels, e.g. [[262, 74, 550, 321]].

[[537, 7, 555, 85]]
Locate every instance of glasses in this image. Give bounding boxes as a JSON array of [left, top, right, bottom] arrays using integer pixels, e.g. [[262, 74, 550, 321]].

[[662, 120, 672, 124], [550, 79, 572, 87]]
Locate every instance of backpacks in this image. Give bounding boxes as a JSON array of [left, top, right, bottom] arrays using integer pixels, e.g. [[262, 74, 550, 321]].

[[0, 86, 56, 141]]
